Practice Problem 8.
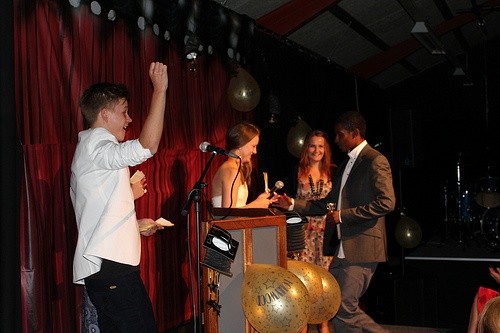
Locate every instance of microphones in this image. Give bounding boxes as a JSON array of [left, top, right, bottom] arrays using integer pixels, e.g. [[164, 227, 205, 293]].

[[267, 180, 284, 199], [199, 141, 238, 158]]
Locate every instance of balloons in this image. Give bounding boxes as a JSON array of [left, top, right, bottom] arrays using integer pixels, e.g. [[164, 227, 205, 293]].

[[287, 117, 312, 159], [242, 260, 342, 333], [227, 66, 260, 112], [396, 212, 422, 249]]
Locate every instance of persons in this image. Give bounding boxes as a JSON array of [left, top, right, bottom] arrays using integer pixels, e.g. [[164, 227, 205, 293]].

[[288, 130, 338, 270], [467, 267, 500, 333], [211, 121, 278, 219], [70, 62, 168, 333], [271, 111, 396, 333]]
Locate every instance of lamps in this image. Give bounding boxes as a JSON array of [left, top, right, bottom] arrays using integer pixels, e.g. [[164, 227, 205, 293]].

[[411, 22, 445, 55], [184, 33, 200, 60]]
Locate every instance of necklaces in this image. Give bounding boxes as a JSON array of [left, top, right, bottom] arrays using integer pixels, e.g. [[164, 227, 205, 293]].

[[308, 175, 323, 200]]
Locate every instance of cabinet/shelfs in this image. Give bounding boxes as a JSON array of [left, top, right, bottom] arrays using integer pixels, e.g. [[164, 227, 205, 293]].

[[201, 207, 288, 333]]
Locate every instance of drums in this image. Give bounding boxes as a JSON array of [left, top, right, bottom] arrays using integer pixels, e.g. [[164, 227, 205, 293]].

[[480, 207, 500, 249], [441, 189, 479, 223], [474, 176, 500, 208]]
